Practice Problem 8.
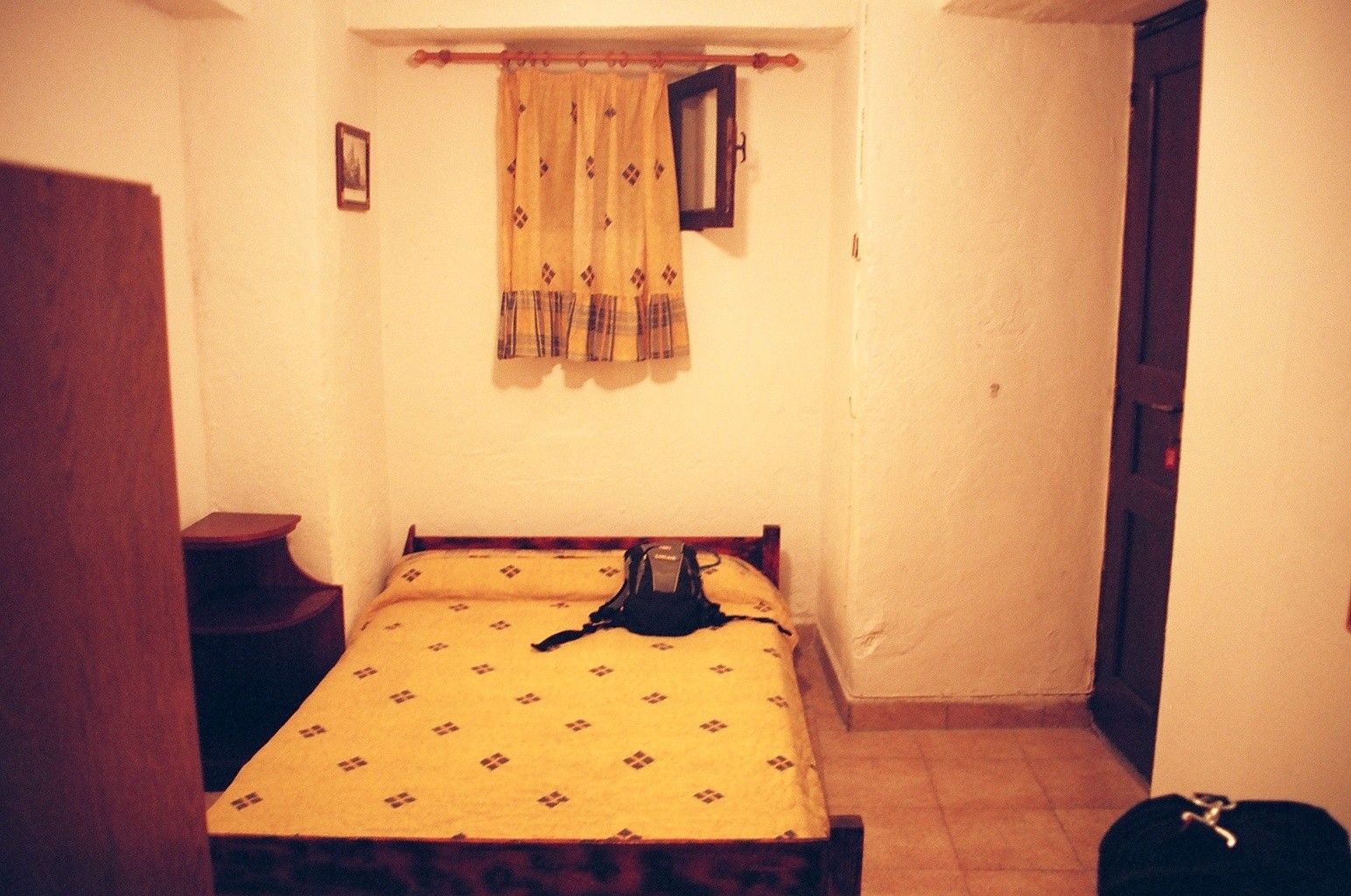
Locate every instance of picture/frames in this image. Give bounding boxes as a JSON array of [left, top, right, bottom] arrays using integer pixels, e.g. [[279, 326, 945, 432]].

[[335, 121, 370, 211]]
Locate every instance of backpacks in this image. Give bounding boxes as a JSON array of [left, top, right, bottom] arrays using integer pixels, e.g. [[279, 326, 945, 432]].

[[590, 540, 729, 635]]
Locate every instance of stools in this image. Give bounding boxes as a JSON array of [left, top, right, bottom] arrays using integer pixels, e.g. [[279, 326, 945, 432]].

[[1100, 796, 1351, 895]]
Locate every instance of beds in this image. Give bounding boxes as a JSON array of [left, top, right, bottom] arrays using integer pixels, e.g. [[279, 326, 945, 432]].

[[202, 523, 863, 896]]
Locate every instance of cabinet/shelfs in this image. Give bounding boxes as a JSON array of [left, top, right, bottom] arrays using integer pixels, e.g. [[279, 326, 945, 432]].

[[182, 511, 347, 793]]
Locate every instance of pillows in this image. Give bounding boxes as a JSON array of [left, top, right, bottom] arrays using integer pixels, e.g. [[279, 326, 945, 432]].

[[343, 549, 798, 650]]
